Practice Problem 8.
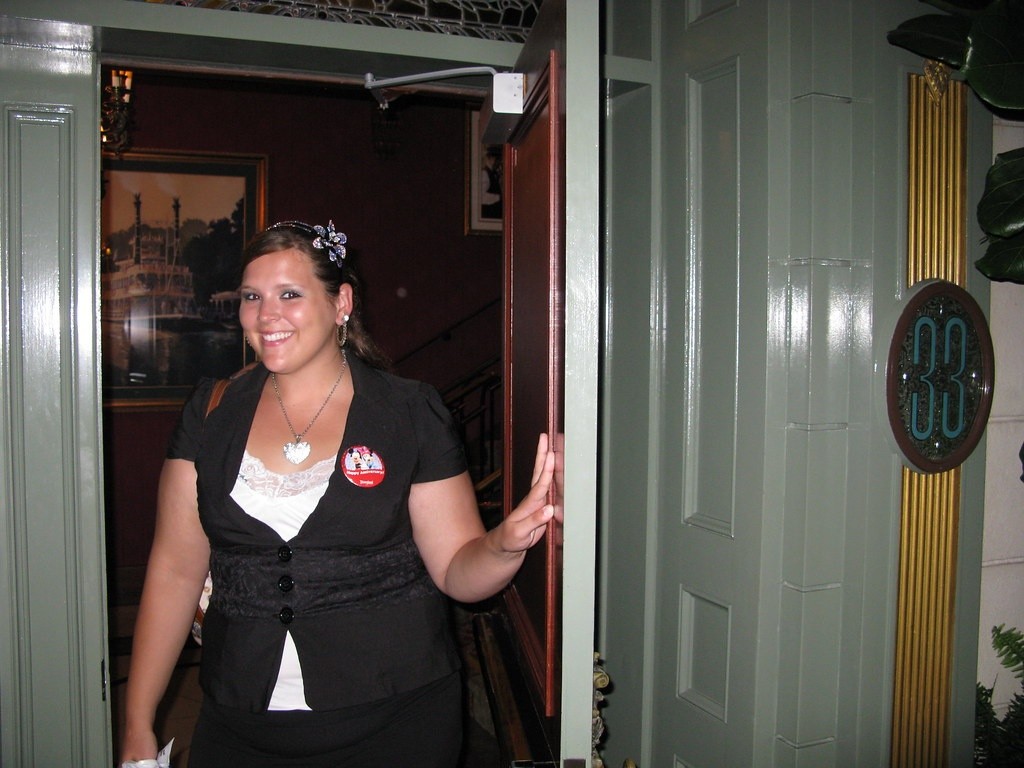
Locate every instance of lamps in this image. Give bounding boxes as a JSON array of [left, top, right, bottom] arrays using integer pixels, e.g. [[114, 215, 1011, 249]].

[[99, 68, 135, 151]]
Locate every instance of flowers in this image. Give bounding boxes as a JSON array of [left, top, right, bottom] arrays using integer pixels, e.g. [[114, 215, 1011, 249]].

[[312, 219, 347, 262]]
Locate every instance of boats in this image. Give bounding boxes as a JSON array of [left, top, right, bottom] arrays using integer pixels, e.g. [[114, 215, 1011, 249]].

[[101, 192, 205, 331]]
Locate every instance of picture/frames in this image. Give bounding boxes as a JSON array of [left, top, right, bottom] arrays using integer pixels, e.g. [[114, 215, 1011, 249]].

[[462, 96, 507, 240], [101, 147, 271, 417]]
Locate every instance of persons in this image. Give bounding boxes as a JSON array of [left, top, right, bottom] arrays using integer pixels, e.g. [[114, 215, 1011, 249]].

[[122, 219, 553, 768]]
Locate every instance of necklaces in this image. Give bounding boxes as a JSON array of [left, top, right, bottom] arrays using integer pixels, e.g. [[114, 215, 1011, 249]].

[[273, 349, 347, 465]]
